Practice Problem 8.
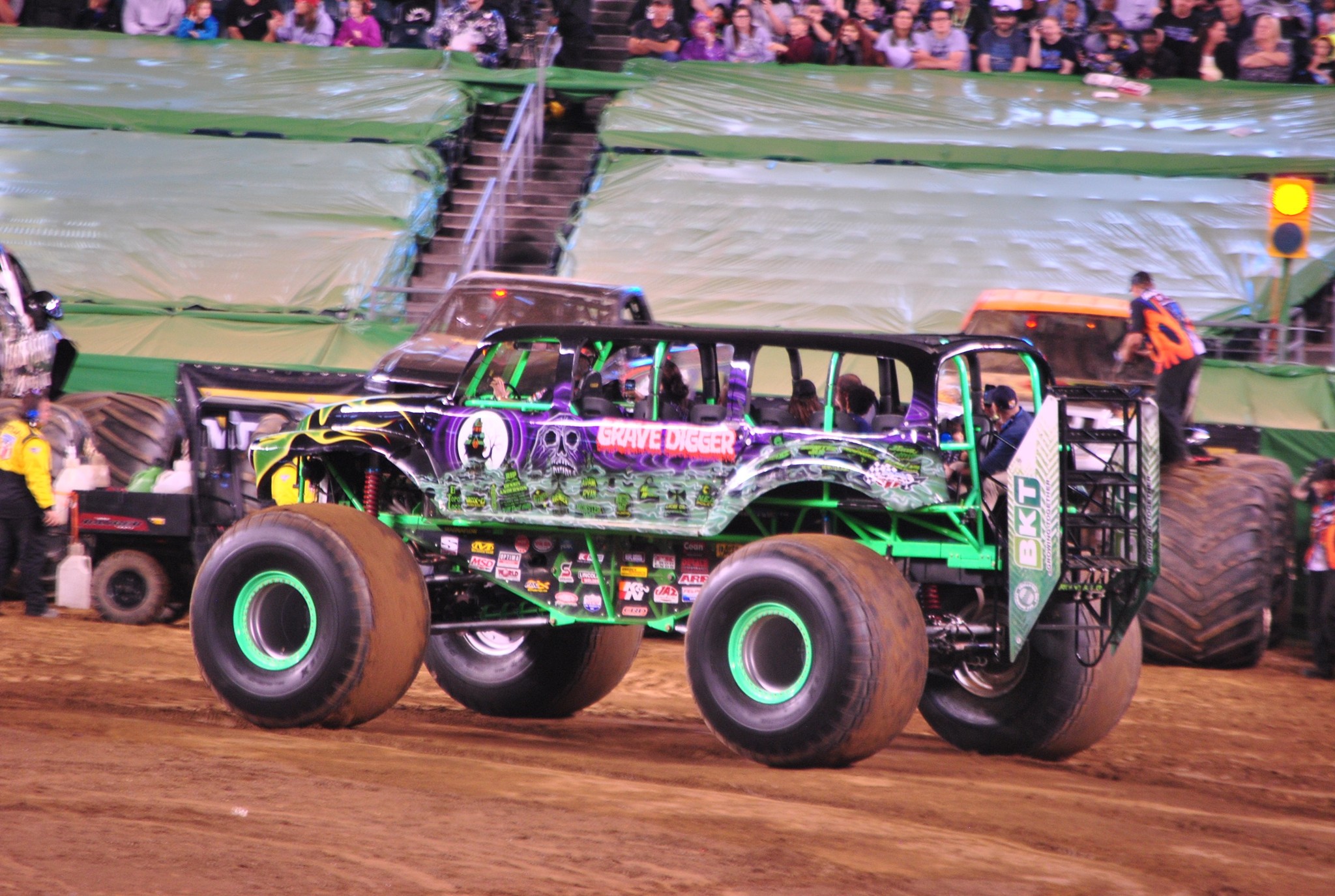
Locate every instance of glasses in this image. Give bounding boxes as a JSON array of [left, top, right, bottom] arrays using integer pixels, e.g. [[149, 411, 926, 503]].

[[929, 16, 951, 22]]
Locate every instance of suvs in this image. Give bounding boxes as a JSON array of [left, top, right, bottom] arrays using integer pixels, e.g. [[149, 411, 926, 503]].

[[361, 268, 657, 404], [1, 246, 180, 606], [925, 289, 1300, 668], [187, 322, 1164, 770]]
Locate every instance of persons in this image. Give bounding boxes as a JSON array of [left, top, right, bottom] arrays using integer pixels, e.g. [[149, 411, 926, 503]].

[[1115, 269, 1207, 469], [1289, 459, 1335, 505], [0, 1, 1335, 83], [480, 349, 1037, 481], [1305, 461, 1335, 680], [1, 390, 69, 619]]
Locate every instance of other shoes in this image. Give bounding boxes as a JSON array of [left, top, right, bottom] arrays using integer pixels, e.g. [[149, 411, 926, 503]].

[[41, 607, 59, 620]]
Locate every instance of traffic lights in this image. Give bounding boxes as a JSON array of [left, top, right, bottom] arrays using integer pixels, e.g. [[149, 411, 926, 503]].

[[1267, 175, 1316, 258]]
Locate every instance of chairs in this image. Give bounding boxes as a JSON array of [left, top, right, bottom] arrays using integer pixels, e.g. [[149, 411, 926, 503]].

[[560, 390, 951, 445]]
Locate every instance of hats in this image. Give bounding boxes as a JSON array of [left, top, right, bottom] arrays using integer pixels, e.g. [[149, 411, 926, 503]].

[[1126, 272, 1156, 294], [1304, 466, 1335, 492], [995, 5, 1017, 19], [792, 380, 816, 400], [987, 385, 1018, 409]]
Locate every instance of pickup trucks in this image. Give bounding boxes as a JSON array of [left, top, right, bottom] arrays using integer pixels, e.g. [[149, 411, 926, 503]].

[[69, 397, 319, 625]]
